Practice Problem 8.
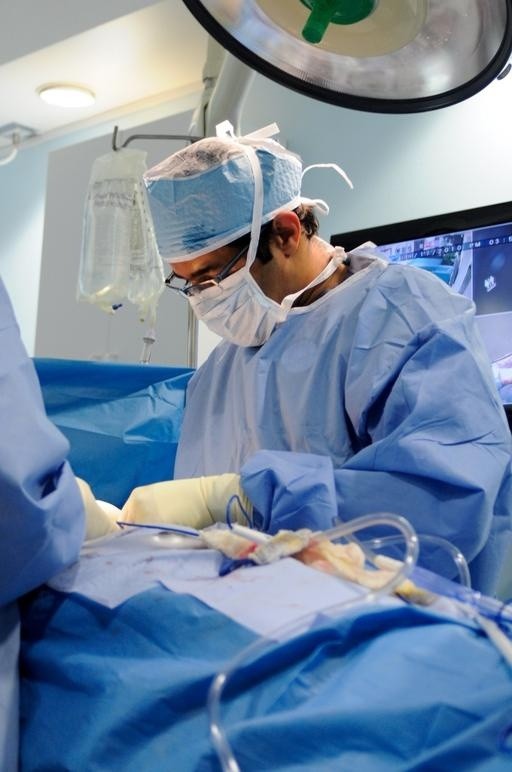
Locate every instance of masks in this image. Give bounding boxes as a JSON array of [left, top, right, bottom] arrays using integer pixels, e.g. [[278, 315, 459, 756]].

[[188, 266, 286, 347]]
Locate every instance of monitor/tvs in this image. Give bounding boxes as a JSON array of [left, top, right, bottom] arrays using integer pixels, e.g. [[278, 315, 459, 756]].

[[331, 201, 512, 433]]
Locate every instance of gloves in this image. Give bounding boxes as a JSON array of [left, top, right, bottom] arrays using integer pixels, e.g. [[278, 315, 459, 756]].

[[75, 477, 123, 543], [120, 472, 255, 532]]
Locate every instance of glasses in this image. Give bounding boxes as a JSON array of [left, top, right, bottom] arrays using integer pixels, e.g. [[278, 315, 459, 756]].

[[164, 246, 248, 300]]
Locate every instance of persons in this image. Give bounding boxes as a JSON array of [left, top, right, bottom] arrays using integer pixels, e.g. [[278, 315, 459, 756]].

[[1, 268, 119, 772], [117, 134, 510, 606]]
